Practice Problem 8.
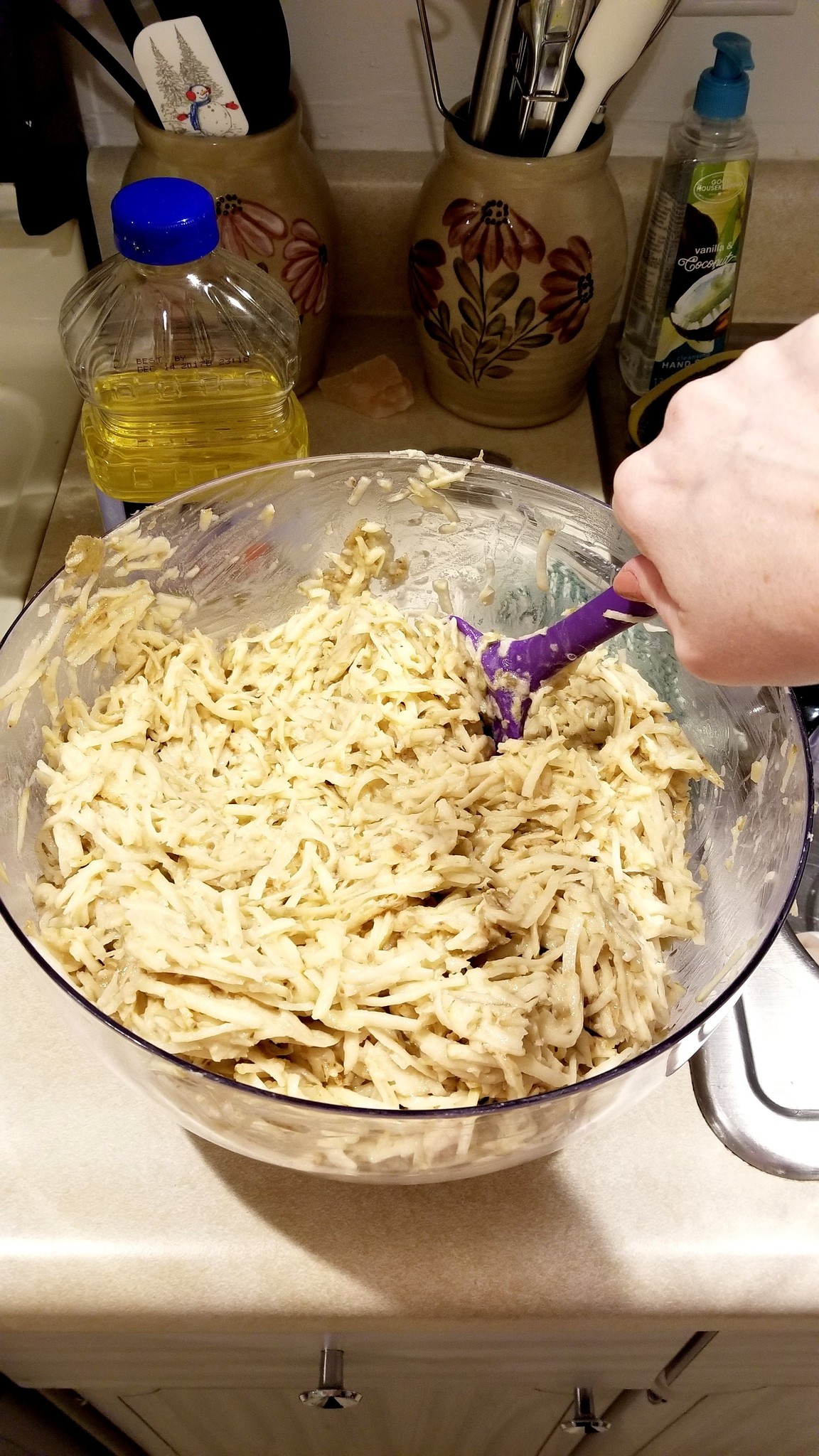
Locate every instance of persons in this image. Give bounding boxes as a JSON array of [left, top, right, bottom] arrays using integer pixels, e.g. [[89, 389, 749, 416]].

[[611, 313, 819, 687]]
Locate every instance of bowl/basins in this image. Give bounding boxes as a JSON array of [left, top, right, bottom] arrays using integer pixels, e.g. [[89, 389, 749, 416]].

[[2, 451, 815, 1187]]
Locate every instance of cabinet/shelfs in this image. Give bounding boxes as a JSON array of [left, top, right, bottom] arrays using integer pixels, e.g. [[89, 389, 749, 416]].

[[0, 1329, 819, 1456]]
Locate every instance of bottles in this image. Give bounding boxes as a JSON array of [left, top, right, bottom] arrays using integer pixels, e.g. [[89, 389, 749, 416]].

[[60, 174, 309, 537], [406, 93, 630, 428], [124, 70, 337, 399]]
